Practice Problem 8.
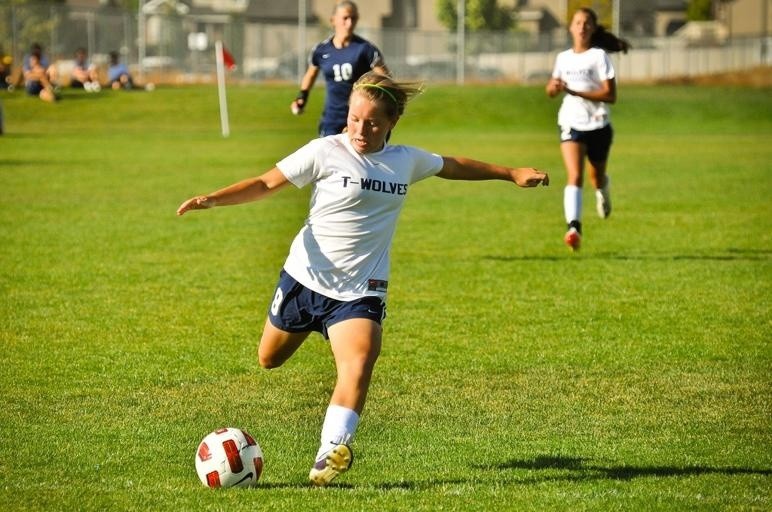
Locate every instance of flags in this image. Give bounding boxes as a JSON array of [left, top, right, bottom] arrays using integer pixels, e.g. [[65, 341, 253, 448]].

[[223, 47, 235, 73]]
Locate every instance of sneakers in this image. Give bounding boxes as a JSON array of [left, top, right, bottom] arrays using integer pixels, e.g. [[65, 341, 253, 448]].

[[307, 442, 352, 487], [565, 231, 580, 251], [595, 191, 612, 217]]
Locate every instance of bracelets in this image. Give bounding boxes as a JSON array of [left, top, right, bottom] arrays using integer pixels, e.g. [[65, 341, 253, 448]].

[[295, 89, 309, 101]]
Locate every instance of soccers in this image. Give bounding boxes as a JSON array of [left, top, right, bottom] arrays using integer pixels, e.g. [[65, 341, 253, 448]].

[[195, 427, 264, 486]]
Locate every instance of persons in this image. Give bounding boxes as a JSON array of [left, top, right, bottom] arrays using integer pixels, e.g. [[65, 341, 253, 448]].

[[0, 40, 136, 105], [175, 66, 552, 491], [546, 7, 635, 252], [290, 0, 396, 145]]
